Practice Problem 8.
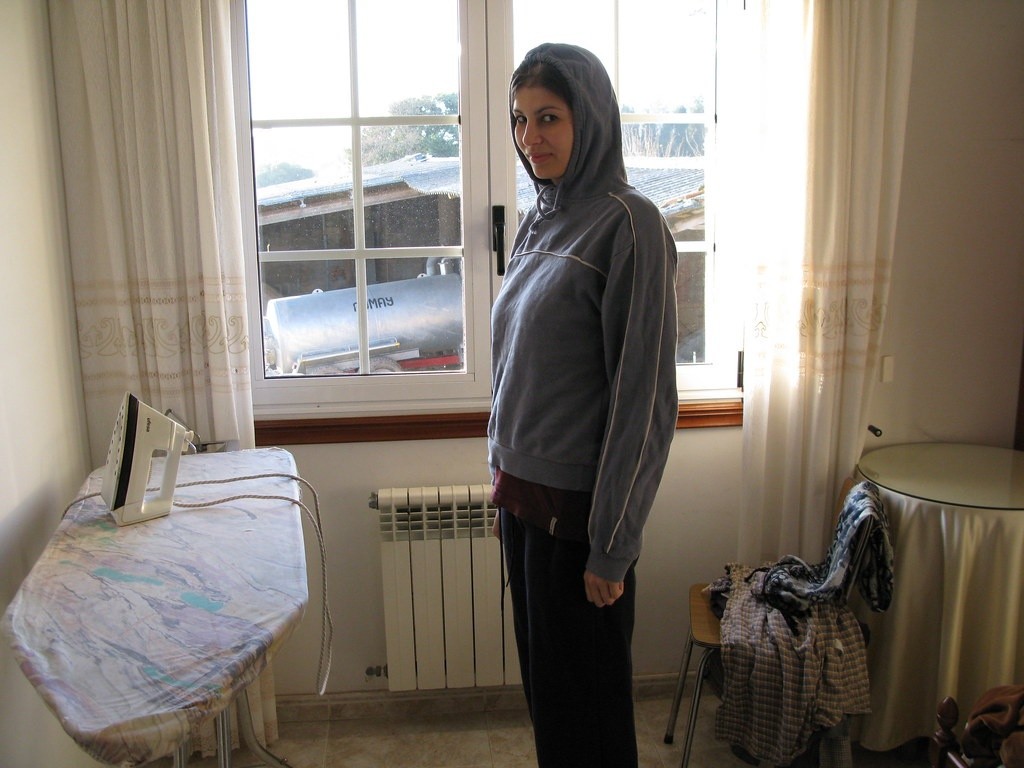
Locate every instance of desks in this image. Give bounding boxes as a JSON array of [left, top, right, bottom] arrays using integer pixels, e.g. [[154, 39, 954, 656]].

[[854, 442, 1024, 768], [0, 447, 310, 768]]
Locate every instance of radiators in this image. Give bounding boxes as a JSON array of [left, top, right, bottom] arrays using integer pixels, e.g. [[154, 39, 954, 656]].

[[368, 484, 524, 691]]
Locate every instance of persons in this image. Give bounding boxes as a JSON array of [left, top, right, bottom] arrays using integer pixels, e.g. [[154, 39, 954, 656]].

[[484, 42, 678, 768]]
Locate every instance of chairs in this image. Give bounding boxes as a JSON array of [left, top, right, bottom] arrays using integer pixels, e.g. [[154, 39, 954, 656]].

[[928, 684, 1024, 768], [663, 478, 895, 768]]
